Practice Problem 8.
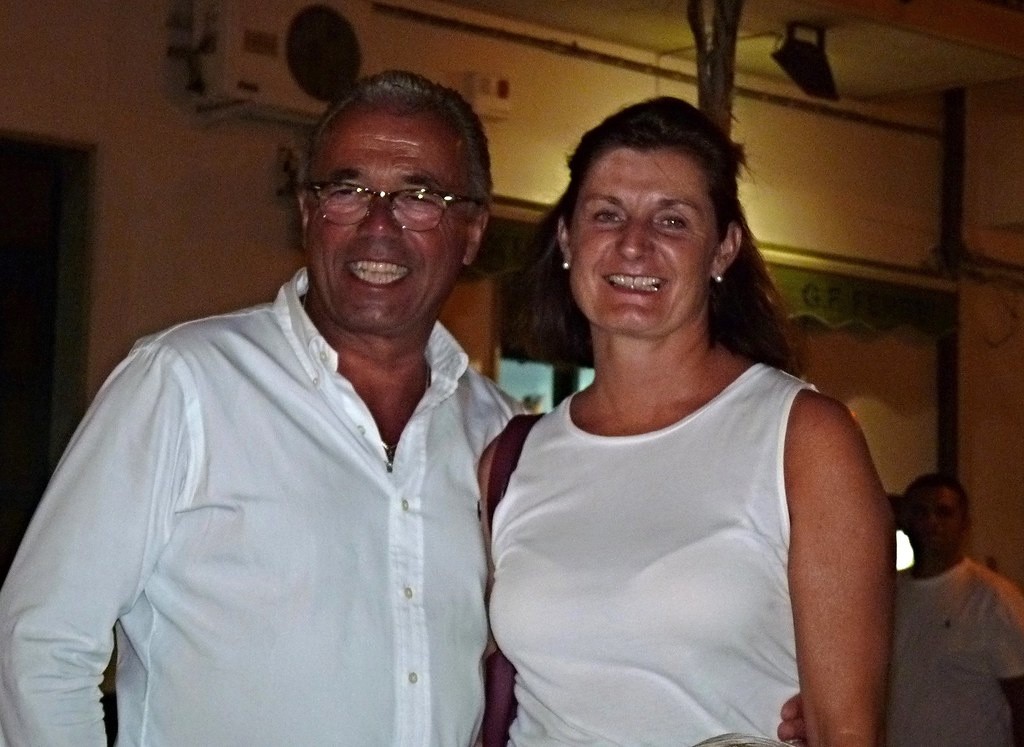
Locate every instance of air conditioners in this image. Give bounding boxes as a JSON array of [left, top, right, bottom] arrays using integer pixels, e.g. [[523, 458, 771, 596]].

[[186, 0, 377, 122]]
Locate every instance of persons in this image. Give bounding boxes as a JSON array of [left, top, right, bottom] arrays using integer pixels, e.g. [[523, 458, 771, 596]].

[[0, 69, 805, 747], [477, 95, 896, 747], [885, 472, 1024, 747]]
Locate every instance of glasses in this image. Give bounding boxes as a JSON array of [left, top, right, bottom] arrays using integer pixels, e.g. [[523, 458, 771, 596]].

[[305, 179, 483, 231]]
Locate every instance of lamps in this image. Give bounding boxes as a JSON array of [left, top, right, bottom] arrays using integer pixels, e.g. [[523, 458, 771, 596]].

[[773, 21, 837, 101]]
